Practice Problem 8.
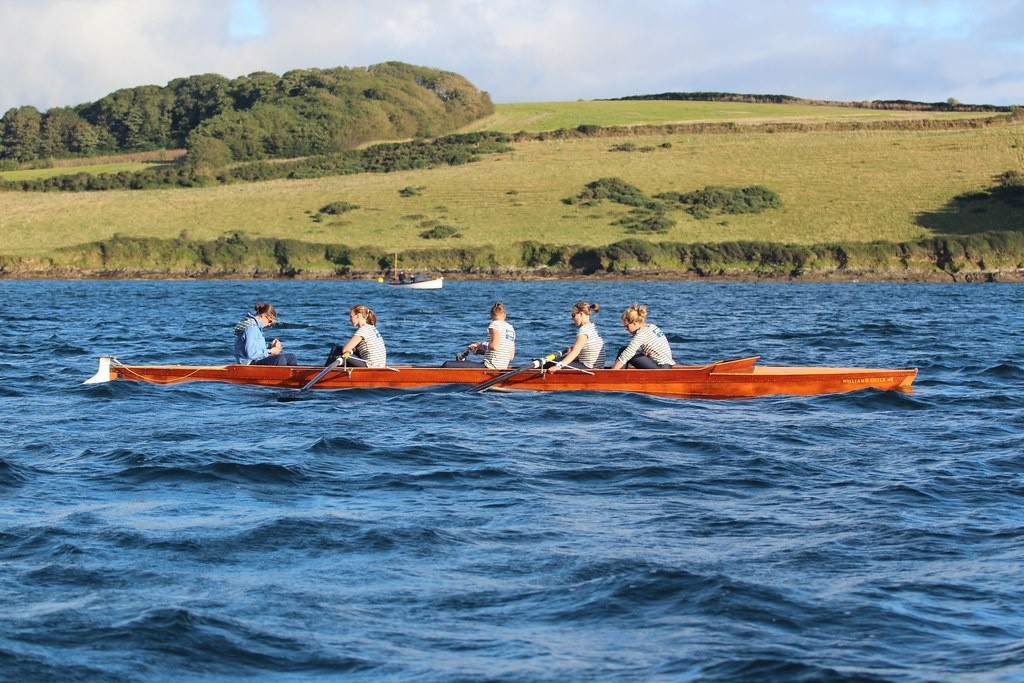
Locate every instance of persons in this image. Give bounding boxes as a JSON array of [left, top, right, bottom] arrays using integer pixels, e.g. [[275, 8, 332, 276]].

[[398, 270, 426, 284], [325, 305, 387, 367], [540, 301, 605, 374], [441, 301, 516, 369], [235, 303, 298, 366], [611, 303, 676, 370]]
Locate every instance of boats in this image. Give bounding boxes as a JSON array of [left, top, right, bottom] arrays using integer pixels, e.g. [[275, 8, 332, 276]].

[[387, 276, 444, 289], [89, 352, 920, 399]]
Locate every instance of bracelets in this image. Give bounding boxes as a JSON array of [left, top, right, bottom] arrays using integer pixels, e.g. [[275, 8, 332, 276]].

[[268, 349, 271, 355], [481, 342, 490, 351], [557, 361, 564, 368]]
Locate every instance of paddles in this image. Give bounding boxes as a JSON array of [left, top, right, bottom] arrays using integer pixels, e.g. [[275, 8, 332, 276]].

[[468, 347, 568, 392], [456, 347, 474, 361], [299, 348, 356, 393]]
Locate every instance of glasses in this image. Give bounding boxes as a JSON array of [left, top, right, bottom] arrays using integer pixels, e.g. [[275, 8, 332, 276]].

[[625, 323, 633, 330], [572, 311, 580, 317], [265, 315, 272, 323]]
